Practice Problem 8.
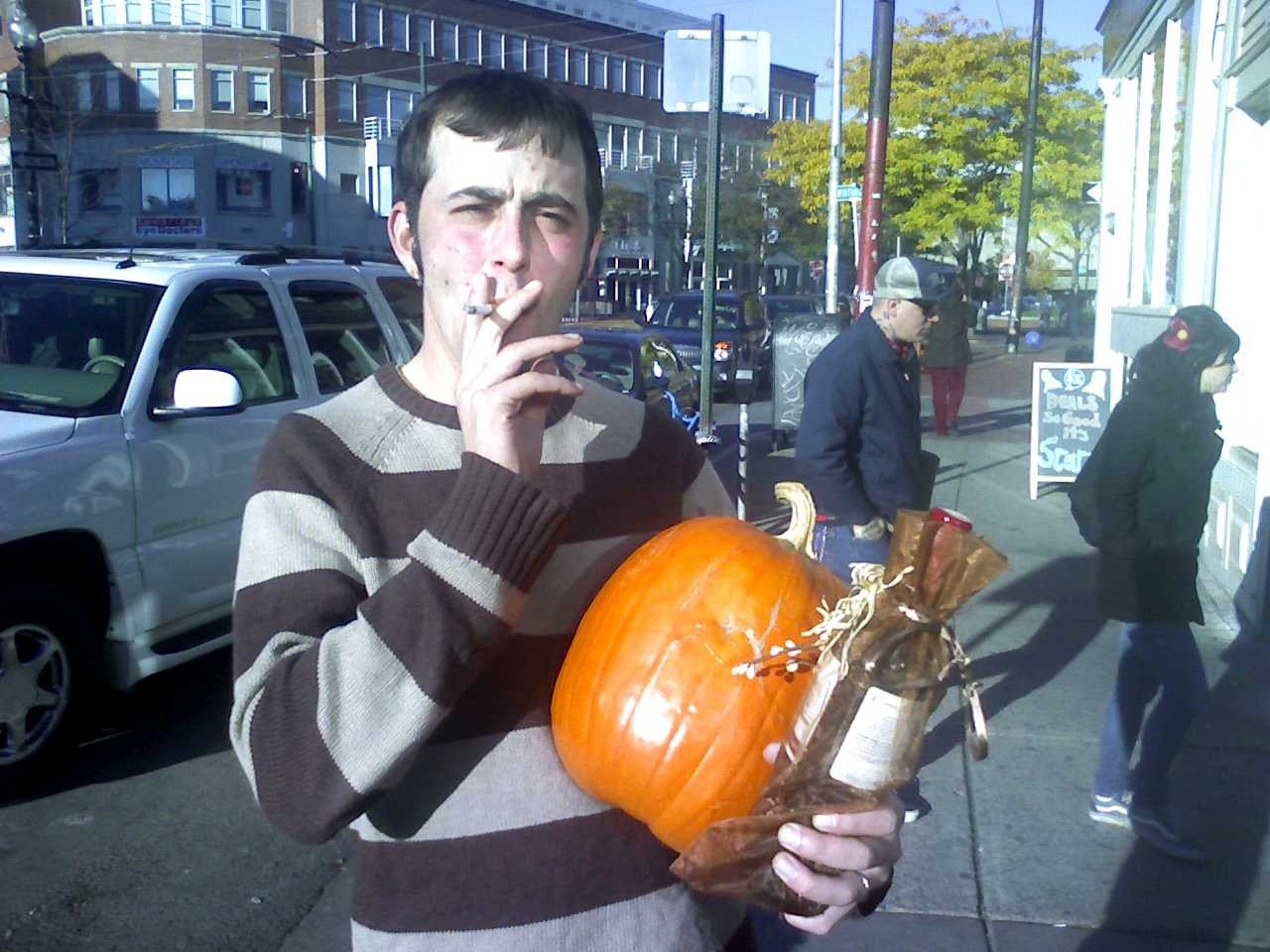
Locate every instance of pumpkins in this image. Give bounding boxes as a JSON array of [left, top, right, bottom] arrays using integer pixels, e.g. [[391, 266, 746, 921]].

[[550, 482, 853, 853]]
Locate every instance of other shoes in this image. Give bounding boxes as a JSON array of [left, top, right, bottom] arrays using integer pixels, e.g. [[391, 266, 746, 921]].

[[903, 807, 920, 823], [945, 420, 960, 435]]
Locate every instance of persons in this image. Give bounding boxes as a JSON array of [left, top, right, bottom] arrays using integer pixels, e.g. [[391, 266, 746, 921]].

[[925, 280, 978, 437], [228, 69, 907, 952], [1068, 306, 1240, 828], [793, 255, 956, 824]]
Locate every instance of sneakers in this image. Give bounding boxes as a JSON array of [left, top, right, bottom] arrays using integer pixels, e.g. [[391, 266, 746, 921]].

[[1088, 785, 1134, 829]]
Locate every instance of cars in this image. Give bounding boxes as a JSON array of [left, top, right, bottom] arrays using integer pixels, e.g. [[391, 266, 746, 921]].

[[559, 320, 701, 441], [758, 292, 856, 327]]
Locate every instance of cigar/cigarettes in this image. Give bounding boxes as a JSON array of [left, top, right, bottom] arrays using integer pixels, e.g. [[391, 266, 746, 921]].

[[466, 304, 497, 316]]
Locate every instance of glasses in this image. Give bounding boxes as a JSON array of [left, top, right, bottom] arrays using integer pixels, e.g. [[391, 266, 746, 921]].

[[907, 299, 942, 318]]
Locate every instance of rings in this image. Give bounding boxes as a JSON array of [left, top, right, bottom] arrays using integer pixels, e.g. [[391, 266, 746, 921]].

[[857, 872, 870, 889]]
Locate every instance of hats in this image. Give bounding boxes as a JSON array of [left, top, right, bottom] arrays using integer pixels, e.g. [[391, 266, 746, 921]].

[[873, 256, 955, 301]]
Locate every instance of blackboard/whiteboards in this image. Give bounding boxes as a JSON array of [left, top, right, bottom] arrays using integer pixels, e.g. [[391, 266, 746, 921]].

[[1031, 361, 1127, 481], [771, 311, 849, 429]]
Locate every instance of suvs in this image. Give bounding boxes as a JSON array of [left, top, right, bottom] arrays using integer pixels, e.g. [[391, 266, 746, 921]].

[[1, 237, 425, 770], [644, 284, 775, 399]]
